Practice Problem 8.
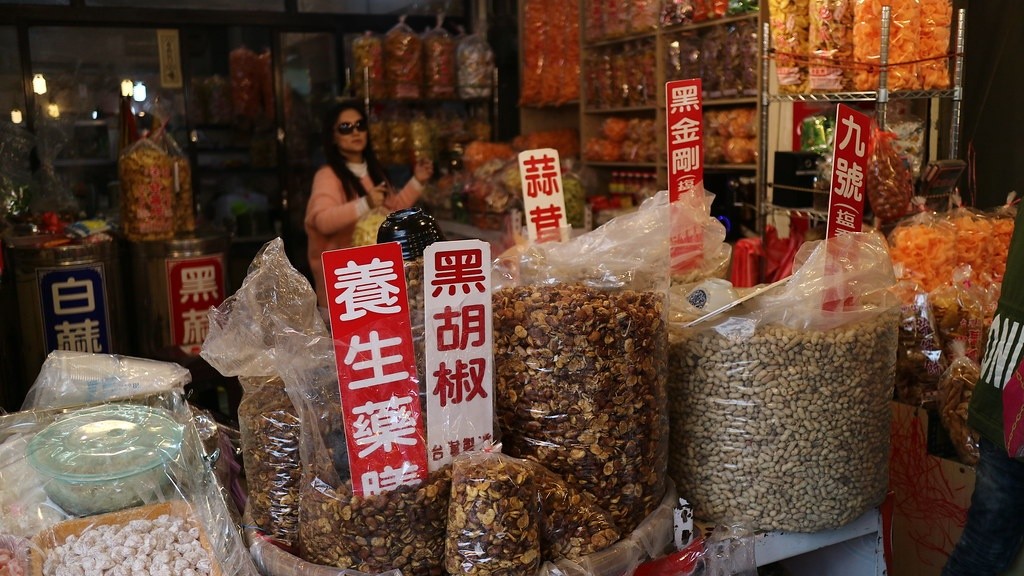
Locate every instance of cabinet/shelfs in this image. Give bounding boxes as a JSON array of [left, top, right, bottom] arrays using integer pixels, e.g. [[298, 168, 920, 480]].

[[338, 0, 967, 252]]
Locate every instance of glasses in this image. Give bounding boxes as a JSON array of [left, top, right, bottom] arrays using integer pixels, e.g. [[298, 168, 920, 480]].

[[337, 117, 369, 135]]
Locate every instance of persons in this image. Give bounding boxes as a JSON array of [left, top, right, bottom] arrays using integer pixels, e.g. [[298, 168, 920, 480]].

[[307, 101, 435, 308]]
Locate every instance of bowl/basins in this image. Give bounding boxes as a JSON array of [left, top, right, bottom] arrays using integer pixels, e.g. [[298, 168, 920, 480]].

[[25, 404, 182, 518], [377, 206, 447, 260]]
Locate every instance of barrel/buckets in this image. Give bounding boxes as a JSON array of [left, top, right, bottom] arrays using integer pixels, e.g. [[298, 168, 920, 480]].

[[135, 233, 232, 363], [14, 243, 124, 378]]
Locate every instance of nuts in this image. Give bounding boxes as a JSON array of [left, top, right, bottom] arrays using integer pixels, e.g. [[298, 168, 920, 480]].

[[667, 309, 896, 533], [241, 283, 668, 576]]
